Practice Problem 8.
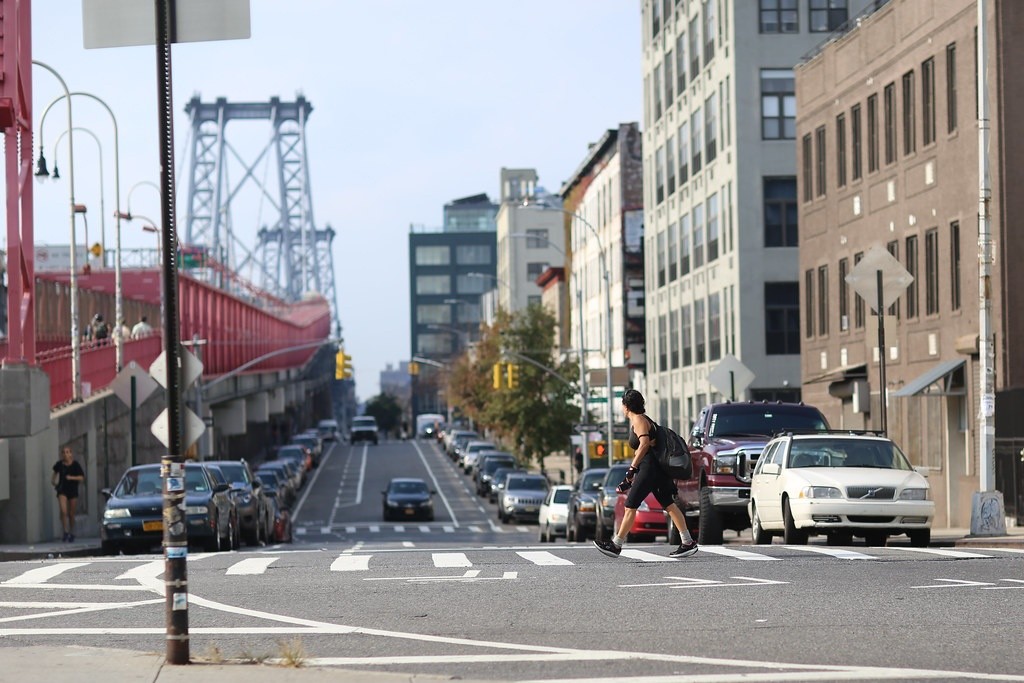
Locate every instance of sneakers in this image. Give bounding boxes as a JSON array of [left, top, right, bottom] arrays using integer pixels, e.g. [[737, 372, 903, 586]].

[[593, 540, 621, 557], [669, 541, 698, 557]]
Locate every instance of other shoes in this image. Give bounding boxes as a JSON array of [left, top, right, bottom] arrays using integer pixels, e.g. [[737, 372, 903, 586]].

[[69, 534, 75, 543], [62, 532, 70, 543]]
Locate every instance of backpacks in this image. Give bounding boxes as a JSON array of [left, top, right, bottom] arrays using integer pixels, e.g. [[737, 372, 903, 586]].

[[644, 415, 692, 480]]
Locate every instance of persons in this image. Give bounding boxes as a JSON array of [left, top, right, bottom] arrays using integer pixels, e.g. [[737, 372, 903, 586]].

[[81, 313, 152, 347], [574, 448, 583, 473], [591, 389, 698, 558], [50, 448, 85, 542]]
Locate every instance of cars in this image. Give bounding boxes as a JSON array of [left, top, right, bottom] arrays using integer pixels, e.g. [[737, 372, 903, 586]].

[[416, 410, 528, 504], [612, 483, 700, 543], [490, 473, 553, 525], [564, 467, 609, 542], [99, 458, 237, 553], [592, 462, 637, 542], [746, 430, 935, 549], [207, 458, 241, 550], [536, 483, 576, 544], [379, 479, 438, 521], [253, 419, 349, 544]]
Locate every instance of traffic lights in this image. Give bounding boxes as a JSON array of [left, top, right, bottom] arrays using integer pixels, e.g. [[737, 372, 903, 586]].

[[507, 363, 519, 390], [589, 439, 610, 458], [336, 350, 353, 380]]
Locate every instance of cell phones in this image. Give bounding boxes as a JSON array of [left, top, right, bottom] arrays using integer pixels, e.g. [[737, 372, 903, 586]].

[[618, 481, 632, 492]]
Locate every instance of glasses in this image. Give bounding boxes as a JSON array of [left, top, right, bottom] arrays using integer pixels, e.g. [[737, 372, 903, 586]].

[[624, 389, 631, 399]]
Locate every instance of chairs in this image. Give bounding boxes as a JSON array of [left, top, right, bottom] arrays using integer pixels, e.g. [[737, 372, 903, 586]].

[[846, 448, 875, 465], [133, 480, 155, 495], [790, 452, 815, 467], [184, 481, 200, 490]]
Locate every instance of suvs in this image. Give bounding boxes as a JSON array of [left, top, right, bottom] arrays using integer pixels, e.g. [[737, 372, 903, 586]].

[[349, 415, 379, 444], [186, 454, 269, 545], [664, 398, 837, 546]]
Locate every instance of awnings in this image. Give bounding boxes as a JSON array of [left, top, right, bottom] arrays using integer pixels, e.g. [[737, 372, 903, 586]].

[[801, 362, 867, 385], [889, 358, 966, 398]]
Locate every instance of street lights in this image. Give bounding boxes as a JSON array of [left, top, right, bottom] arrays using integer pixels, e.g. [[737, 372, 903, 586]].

[[35, 90, 126, 375], [516, 201, 617, 469], [465, 270, 523, 312], [53, 126, 107, 269]]
